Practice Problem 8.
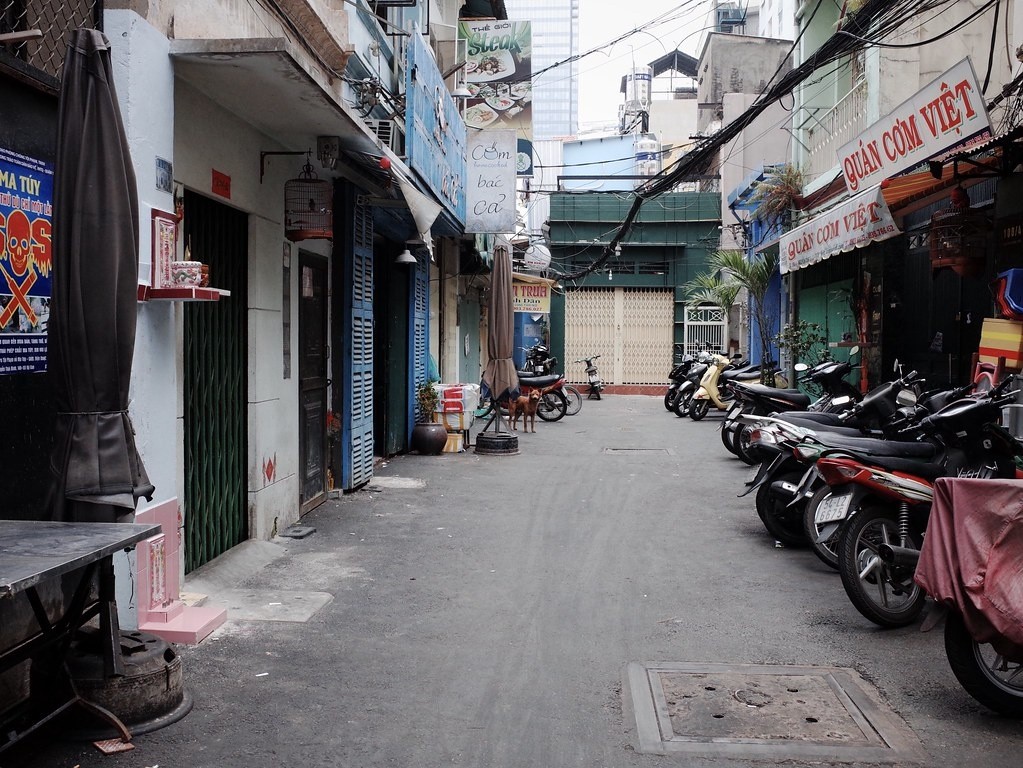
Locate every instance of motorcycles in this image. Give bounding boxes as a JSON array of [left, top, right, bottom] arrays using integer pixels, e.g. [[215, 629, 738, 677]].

[[665, 340, 1023, 717], [474, 338, 582, 422], [574, 354, 606, 400]]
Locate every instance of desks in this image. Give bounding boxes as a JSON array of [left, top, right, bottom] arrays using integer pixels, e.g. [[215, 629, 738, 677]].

[[0, 518, 161, 751]]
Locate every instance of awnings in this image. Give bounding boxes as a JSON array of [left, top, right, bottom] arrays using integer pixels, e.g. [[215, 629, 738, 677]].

[[513, 273, 555, 288], [779, 158, 997, 274]]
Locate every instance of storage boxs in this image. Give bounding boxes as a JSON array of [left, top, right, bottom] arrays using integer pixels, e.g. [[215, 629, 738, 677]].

[[443, 434, 464, 454], [433, 411, 474, 431], [431, 384, 479, 412]]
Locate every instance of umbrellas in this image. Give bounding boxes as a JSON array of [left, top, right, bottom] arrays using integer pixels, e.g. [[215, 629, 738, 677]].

[[31, 30, 196, 735], [472, 244, 518, 455]]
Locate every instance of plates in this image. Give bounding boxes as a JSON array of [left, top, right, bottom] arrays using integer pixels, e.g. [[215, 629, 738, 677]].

[[460, 82, 532, 129], [466, 49, 516, 83]]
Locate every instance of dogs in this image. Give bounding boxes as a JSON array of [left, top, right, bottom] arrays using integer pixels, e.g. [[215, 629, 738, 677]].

[[508, 387, 541, 433]]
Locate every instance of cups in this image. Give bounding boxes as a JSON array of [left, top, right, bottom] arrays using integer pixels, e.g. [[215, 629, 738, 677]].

[[199, 264, 209, 288], [171, 261, 202, 287]]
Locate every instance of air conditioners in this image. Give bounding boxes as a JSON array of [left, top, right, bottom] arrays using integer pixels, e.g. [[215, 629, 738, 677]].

[[364, 118, 405, 162]]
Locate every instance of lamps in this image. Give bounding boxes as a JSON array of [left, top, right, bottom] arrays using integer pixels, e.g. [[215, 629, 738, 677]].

[[450, 82, 472, 100], [395, 224, 418, 264]]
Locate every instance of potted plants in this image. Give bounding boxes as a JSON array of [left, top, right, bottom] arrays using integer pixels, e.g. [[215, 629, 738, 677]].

[[412, 380, 448, 455]]
